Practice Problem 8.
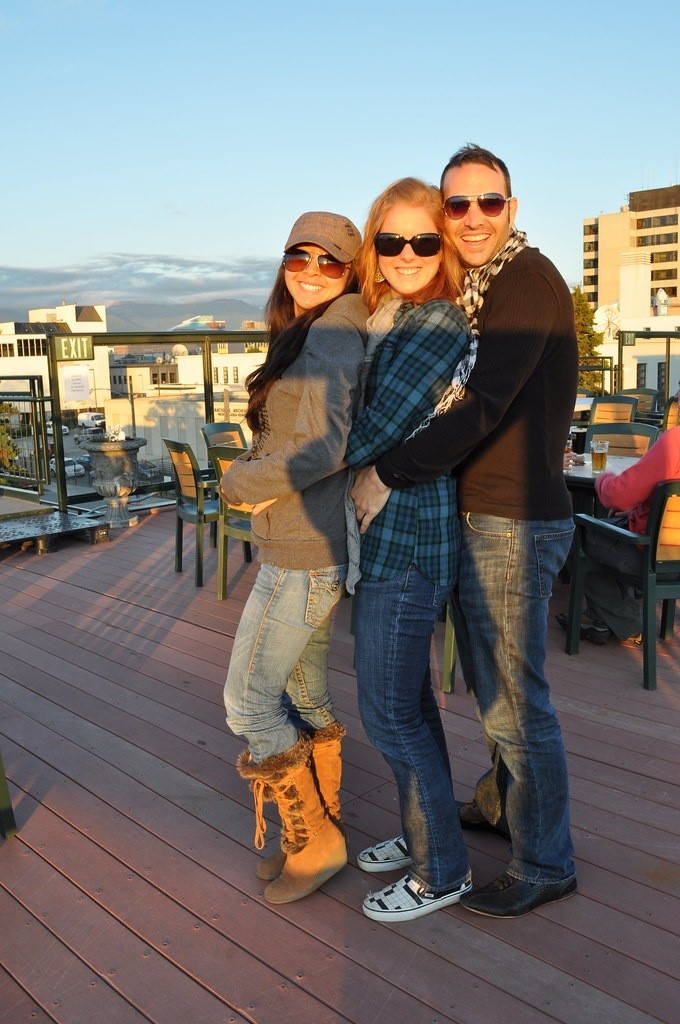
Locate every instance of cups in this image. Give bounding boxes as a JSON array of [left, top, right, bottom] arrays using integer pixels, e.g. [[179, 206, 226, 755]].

[[562, 440, 573, 473], [590, 441, 610, 473], [571, 428, 587, 466]]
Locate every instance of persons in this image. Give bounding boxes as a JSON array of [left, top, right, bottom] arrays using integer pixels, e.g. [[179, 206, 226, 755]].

[[214, 146, 578, 925], [564, 451, 577, 470], [556, 419, 680, 645]]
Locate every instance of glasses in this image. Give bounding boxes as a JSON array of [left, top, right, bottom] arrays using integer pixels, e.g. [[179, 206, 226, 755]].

[[284, 249, 351, 280], [373, 232, 442, 257], [441, 192, 513, 220]]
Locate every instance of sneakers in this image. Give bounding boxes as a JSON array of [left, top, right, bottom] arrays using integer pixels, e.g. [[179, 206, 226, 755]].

[[454, 798, 513, 841], [555, 611, 610, 645], [460, 871, 578, 919], [618, 632, 644, 649]]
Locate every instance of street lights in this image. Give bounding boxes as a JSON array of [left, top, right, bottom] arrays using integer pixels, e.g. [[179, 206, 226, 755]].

[[88, 376, 136, 438]]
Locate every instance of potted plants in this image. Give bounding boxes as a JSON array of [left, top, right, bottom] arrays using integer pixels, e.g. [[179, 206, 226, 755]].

[[78, 424, 147, 529]]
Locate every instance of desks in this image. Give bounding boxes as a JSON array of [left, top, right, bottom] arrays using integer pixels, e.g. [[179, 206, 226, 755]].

[[562, 453, 641, 520]]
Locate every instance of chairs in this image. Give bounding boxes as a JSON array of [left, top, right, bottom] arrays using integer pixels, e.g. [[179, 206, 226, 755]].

[[161, 389, 680, 692]]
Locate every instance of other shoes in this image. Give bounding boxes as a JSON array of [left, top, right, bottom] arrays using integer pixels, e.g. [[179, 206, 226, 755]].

[[357, 833, 414, 872], [362, 866, 473, 923]]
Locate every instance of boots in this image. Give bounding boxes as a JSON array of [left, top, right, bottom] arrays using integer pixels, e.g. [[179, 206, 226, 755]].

[[236, 731, 348, 904], [254, 720, 346, 880]]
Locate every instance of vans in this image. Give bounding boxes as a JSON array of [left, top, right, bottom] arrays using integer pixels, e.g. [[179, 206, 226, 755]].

[[78, 411, 105, 429]]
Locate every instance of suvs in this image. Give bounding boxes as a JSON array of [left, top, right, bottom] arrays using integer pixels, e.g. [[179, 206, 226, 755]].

[[73, 427, 105, 446]]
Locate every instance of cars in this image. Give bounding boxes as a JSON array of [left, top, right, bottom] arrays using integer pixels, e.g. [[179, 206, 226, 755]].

[[74, 453, 92, 473], [46, 420, 70, 436], [49, 456, 86, 481], [137, 460, 163, 483], [39, 441, 55, 454]]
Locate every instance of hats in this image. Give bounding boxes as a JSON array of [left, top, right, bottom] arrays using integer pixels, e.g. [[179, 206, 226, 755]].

[[284, 211, 363, 264]]
